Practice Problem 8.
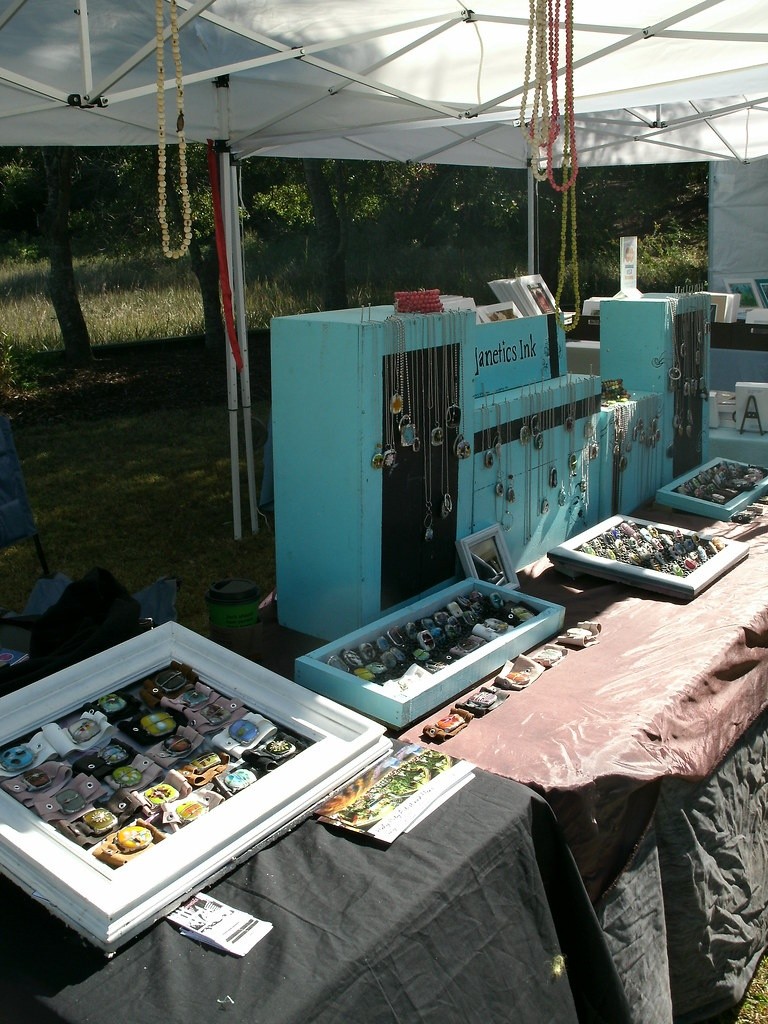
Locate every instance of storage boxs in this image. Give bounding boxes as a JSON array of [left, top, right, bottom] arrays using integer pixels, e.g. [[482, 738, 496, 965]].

[[292, 578, 566, 725], [655, 456, 768, 521], [546, 513, 751, 599], [708, 382, 768, 432]]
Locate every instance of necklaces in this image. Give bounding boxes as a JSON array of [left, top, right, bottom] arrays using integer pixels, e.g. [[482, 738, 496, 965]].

[[665, 295, 710, 436], [607, 393, 661, 471], [367, 310, 598, 535]]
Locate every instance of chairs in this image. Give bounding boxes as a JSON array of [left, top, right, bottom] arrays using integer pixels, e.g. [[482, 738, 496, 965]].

[[0, 415, 181, 633]]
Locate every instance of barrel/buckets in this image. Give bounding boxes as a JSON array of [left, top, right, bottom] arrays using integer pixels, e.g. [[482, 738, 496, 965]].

[[203, 576, 264, 629]]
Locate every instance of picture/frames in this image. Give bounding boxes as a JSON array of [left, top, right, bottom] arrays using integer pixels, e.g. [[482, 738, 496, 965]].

[[698, 277, 768, 324], [454, 523, 520, 589], [440, 274, 563, 324]]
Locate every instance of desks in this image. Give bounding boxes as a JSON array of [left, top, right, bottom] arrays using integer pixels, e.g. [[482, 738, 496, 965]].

[[0, 502, 768, 1024]]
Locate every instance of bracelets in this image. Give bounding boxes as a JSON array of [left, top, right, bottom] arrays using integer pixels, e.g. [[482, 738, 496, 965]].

[[601, 379, 631, 401], [394, 289, 444, 313]]
